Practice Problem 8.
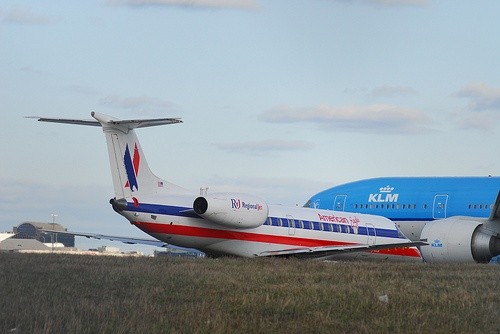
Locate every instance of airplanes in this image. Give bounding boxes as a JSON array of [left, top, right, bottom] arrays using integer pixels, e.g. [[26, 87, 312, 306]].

[[34, 174, 500, 265], [22, 112, 430, 263]]
